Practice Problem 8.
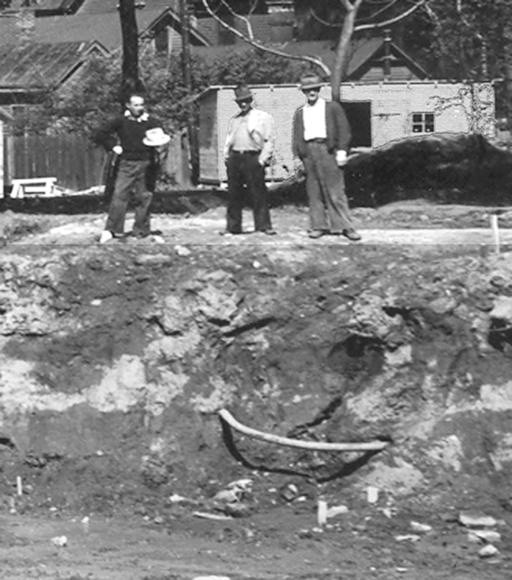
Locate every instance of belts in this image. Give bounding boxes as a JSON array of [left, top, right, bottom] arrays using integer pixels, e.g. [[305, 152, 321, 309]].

[[230, 148, 260, 155], [305, 137, 327, 144]]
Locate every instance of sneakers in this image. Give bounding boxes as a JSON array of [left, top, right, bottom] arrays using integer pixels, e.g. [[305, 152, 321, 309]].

[[99, 230, 167, 245], [309, 229, 326, 238], [218, 226, 277, 236], [341, 227, 362, 241]]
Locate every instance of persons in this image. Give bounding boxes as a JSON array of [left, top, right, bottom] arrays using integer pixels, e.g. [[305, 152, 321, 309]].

[[290, 73, 363, 241], [88, 91, 172, 242], [217, 82, 280, 237]]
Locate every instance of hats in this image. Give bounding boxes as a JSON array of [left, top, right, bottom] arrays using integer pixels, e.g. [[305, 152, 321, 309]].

[[297, 71, 326, 90], [233, 83, 255, 102], [142, 127, 173, 147]]
